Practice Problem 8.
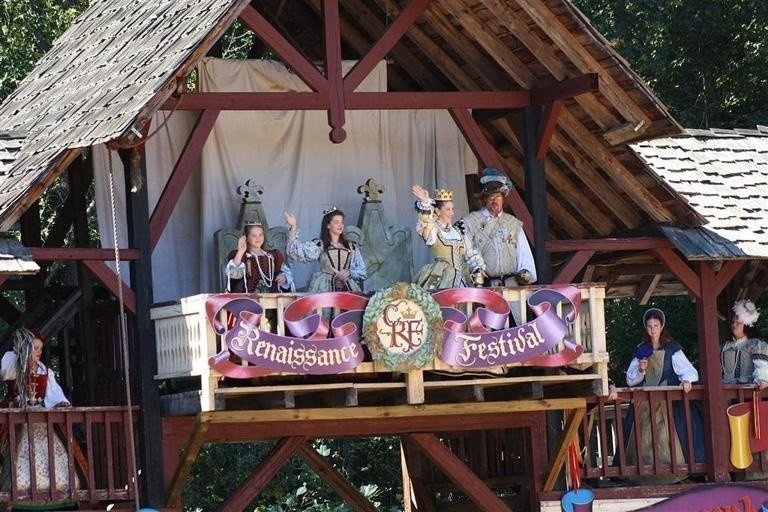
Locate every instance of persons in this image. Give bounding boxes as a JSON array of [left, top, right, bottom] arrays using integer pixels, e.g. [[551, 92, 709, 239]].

[[461, 191, 537, 288], [719, 298, 768, 391], [0, 327, 71, 492], [282, 209, 367, 293], [409, 184, 485, 287], [224, 221, 292, 292], [625, 308, 699, 485]]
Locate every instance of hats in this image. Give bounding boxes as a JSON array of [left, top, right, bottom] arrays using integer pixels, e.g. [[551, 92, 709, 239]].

[[480, 166, 513, 195], [643, 308, 664, 331]]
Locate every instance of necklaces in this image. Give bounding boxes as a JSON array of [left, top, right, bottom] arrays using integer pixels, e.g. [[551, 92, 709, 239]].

[[721, 346, 740, 376], [248, 248, 275, 288]]
